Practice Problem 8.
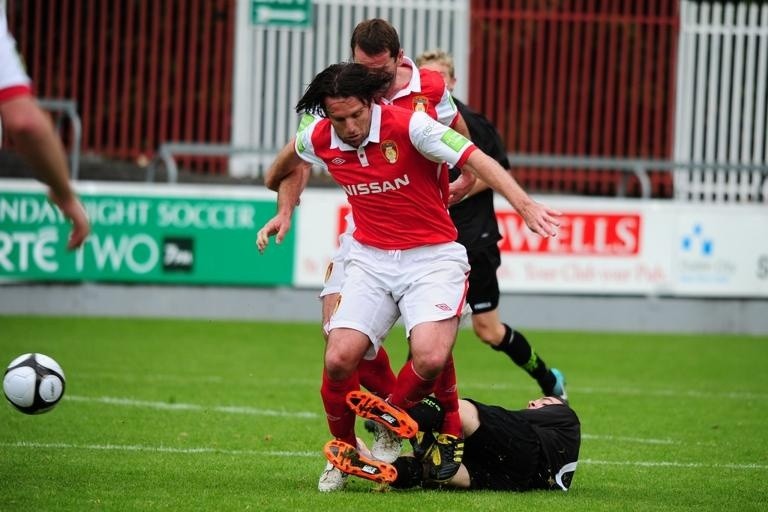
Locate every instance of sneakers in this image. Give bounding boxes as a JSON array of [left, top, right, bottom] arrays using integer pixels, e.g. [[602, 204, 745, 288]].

[[346, 389, 419, 438], [325, 442, 396, 484], [317, 438, 363, 493], [410, 429, 439, 459], [373, 421, 400, 459], [363, 421, 402, 447], [432, 435, 465, 482], [542, 366, 570, 408]]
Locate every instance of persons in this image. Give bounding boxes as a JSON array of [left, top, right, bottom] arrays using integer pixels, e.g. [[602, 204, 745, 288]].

[[0, 1, 93, 250], [321, 390, 583, 494], [262, 60, 567, 491], [251, 17, 481, 490], [401, 46, 568, 418]]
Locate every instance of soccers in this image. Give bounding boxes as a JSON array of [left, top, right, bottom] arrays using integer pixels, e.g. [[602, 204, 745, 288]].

[[3, 353, 65, 414]]
